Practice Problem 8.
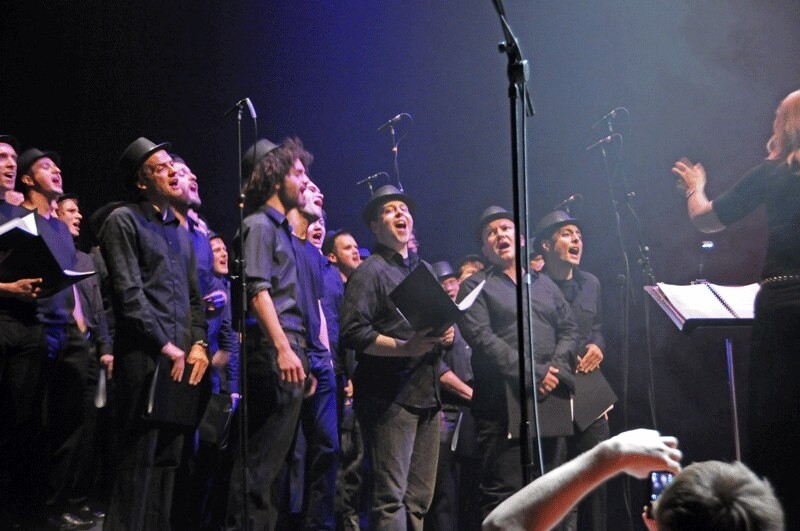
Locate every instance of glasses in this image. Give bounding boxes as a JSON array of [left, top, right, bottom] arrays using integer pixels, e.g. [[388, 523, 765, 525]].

[[441, 279, 459, 289]]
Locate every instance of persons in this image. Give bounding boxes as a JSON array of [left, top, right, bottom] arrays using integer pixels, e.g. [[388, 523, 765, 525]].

[[482, 429, 785, 531], [0, 134, 613, 531], [671, 90, 800, 531]]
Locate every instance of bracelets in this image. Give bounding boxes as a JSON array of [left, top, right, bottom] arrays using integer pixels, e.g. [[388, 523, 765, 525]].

[[686, 187, 705, 198], [195, 340, 208, 349]]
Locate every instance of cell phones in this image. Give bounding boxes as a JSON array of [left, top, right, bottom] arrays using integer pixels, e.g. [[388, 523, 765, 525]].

[[647, 471, 675, 519]]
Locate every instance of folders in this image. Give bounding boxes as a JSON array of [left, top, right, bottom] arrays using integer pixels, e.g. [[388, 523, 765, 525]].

[[143, 357, 208, 428], [92, 362, 114, 416], [643, 280, 759, 330], [502, 380, 574, 439], [449, 405, 478, 454], [573, 366, 619, 433], [0, 226, 100, 297], [198, 392, 239, 449], [388, 261, 487, 337]]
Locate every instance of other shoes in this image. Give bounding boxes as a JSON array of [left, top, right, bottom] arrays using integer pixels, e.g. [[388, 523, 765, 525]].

[[46, 512, 96, 531], [74, 506, 105, 520]]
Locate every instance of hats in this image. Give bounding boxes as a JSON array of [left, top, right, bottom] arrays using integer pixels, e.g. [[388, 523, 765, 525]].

[[57, 191, 78, 203], [431, 260, 463, 279], [474, 206, 514, 248], [0, 134, 19, 153], [15, 149, 56, 176], [240, 138, 286, 189], [322, 228, 343, 254], [533, 210, 579, 253], [363, 184, 416, 229], [205, 226, 220, 240], [114, 137, 172, 193]]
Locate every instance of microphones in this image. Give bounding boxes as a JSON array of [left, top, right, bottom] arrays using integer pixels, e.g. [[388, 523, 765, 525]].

[[586, 134, 615, 150], [376, 113, 405, 134], [593, 108, 618, 130], [224, 97, 247, 117], [356, 171, 387, 186]]
